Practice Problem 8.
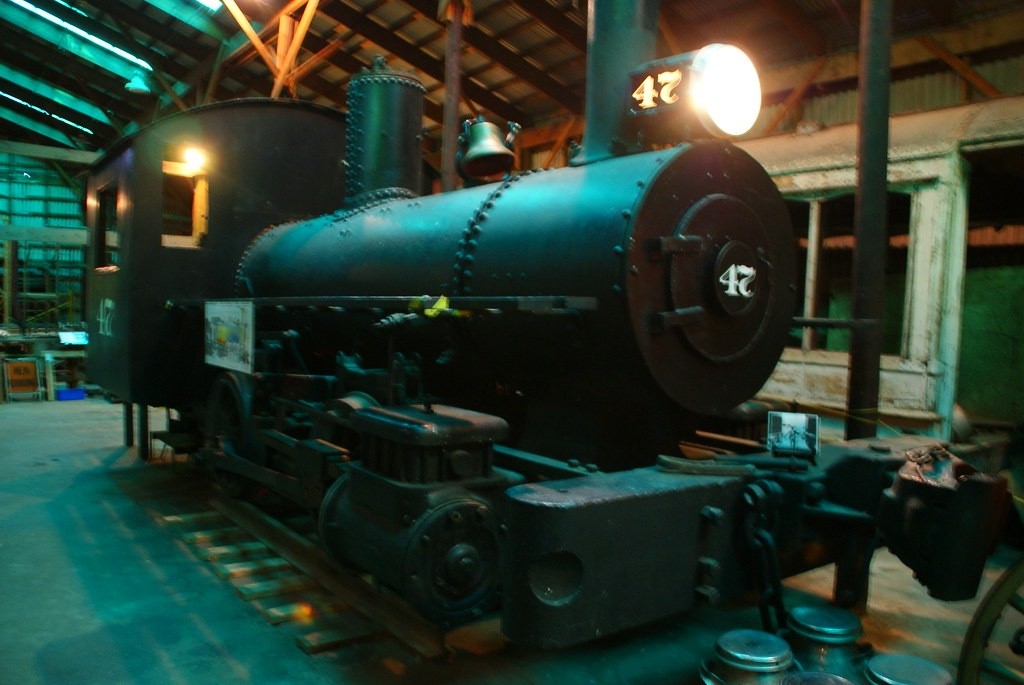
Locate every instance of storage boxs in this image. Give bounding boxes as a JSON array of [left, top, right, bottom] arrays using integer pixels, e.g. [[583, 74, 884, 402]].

[[56, 386, 87, 401]]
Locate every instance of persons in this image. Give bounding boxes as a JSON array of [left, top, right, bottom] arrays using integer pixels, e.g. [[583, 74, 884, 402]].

[[785, 427, 800, 448]]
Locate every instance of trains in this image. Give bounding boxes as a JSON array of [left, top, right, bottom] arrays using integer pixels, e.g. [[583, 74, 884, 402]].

[[80, 44, 1009, 654]]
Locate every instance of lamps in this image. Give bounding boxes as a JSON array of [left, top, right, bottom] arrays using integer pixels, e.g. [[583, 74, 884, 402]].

[[125, 71, 151, 94]]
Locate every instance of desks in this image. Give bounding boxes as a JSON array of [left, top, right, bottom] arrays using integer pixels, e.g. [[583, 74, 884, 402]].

[[40, 350, 101, 401]]
